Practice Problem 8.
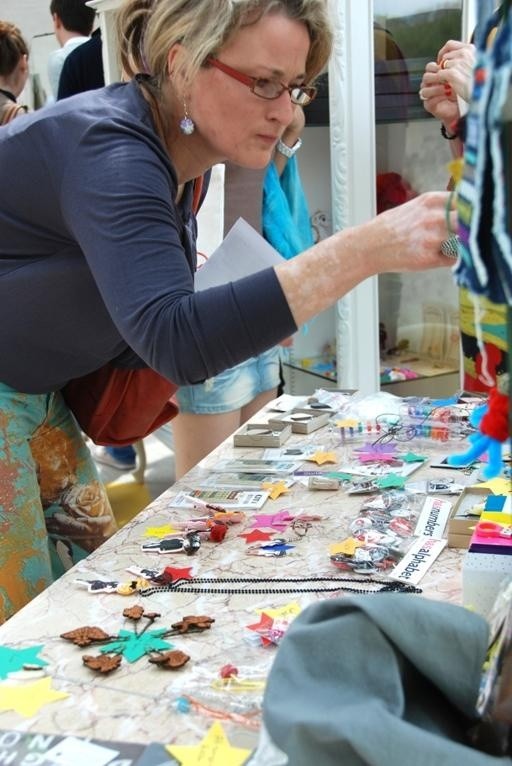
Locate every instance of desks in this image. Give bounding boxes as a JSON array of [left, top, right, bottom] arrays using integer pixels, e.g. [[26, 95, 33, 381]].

[[0, 395, 512, 766]]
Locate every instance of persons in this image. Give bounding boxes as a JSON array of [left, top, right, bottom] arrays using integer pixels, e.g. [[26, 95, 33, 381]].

[[415, 36, 479, 162], [0, 0, 459, 629], [44, 0, 104, 108], [0, 17, 30, 128], [172, 89, 307, 484]]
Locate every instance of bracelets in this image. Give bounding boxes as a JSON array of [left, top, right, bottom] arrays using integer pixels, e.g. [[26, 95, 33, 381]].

[[439, 121, 459, 140], [277, 137, 304, 158]]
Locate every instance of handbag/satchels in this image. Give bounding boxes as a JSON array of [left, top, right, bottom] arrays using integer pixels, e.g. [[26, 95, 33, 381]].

[[61, 363, 178, 447]]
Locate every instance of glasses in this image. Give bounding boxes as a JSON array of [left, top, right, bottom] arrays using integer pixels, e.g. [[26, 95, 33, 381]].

[[206, 54, 318, 106]]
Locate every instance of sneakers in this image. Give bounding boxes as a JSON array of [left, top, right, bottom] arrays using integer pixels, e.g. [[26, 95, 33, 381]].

[[90, 446, 136, 470]]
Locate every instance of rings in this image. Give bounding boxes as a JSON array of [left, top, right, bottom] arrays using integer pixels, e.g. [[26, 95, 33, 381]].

[[419, 93, 430, 103], [439, 57, 452, 69], [443, 83, 452, 98]]
